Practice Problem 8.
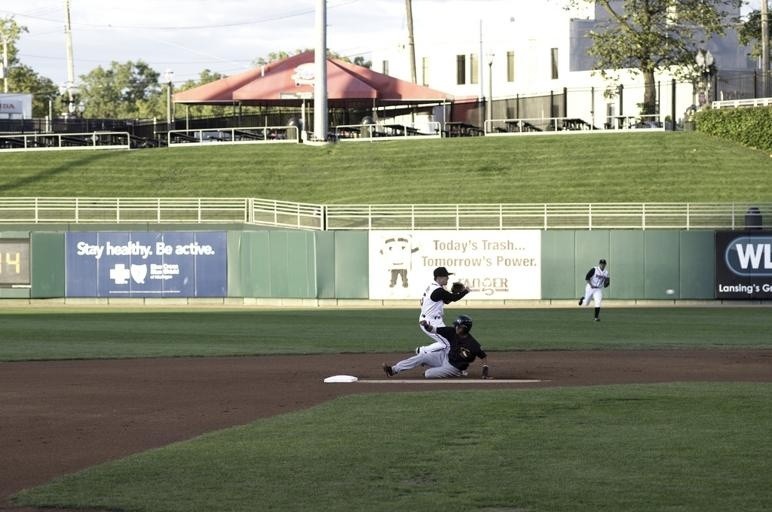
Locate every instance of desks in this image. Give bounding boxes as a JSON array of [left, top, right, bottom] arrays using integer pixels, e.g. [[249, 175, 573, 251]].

[[0, 128, 264, 148], [333, 116, 594, 137]]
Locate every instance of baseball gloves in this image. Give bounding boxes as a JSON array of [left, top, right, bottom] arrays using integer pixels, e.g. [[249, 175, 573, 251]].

[[604, 277, 609, 288], [451, 283, 464, 294]]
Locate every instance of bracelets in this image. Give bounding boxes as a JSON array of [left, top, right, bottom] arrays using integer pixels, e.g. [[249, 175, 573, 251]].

[[482, 364, 488, 368], [424, 324, 429, 329]]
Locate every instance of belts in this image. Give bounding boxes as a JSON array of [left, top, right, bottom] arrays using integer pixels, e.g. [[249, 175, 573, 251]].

[[422, 315, 440, 320]]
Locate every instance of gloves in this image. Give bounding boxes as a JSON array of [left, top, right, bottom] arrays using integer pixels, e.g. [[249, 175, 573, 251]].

[[417, 320, 428, 328], [480, 365, 489, 379]]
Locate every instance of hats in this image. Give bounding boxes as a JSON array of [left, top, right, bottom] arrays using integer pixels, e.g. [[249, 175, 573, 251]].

[[599, 259, 607, 265], [433, 266, 454, 277]]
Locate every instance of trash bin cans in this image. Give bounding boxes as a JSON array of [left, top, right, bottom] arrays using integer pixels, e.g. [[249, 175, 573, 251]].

[[745, 208, 762, 230], [361, 116, 372, 137], [286, 118, 300, 139]]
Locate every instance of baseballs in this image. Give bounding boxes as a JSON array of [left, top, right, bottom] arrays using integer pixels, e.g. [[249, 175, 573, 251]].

[[667, 290, 675, 295]]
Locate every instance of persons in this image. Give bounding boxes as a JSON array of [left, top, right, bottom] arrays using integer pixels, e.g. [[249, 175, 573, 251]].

[[415, 266, 470, 368], [380, 314, 489, 380], [579, 258, 611, 321]]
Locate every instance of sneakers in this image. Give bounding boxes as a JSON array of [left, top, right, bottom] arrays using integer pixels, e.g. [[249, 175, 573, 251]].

[[578, 295, 601, 322], [381, 362, 393, 378], [415, 346, 425, 368]]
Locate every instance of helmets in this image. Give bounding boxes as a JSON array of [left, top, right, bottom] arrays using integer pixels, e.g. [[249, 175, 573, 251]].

[[454, 315, 472, 331]]
